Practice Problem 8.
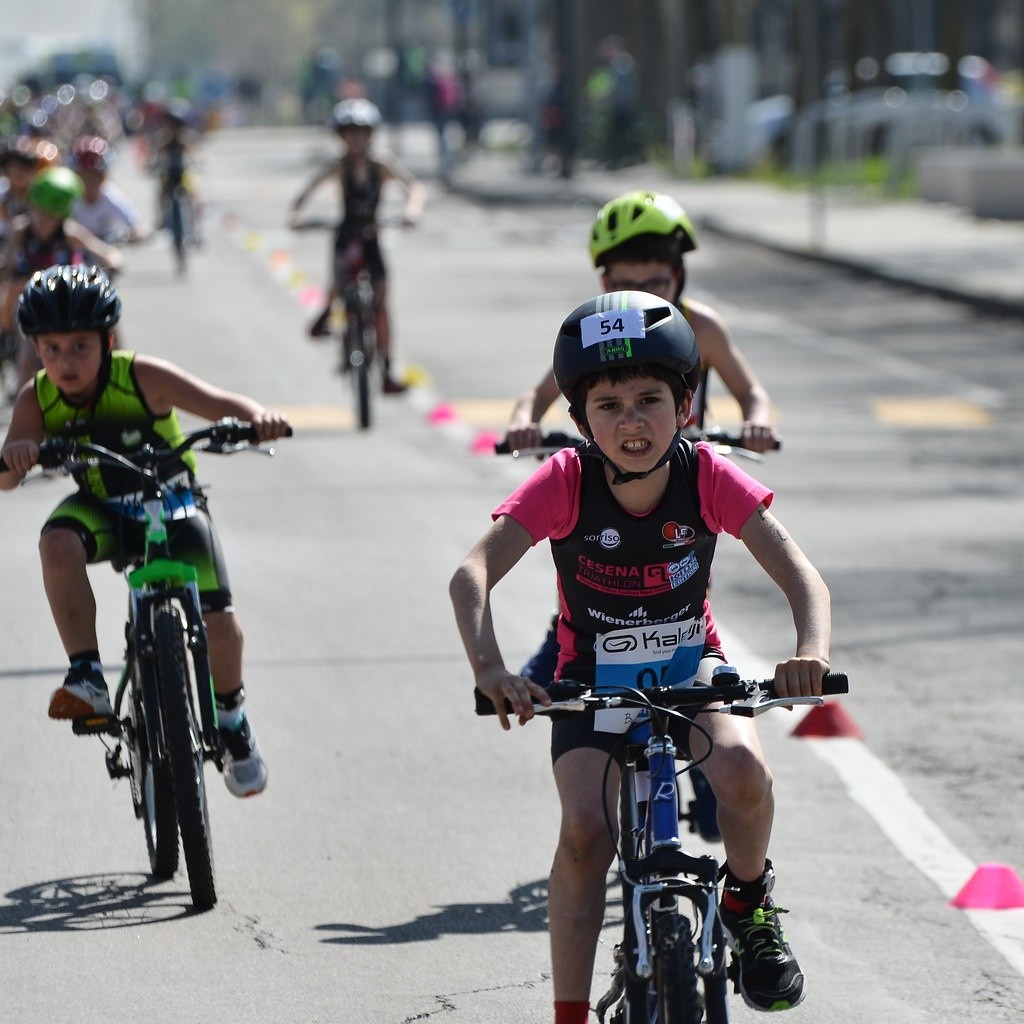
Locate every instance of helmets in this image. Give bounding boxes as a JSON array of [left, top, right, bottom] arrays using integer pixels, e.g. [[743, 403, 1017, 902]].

[[18, 262, 124, 340], [332, 99, 381, 137], [3, 129, 112, 215], [591, 189, 697, 267], [551, 291, 700, 397]]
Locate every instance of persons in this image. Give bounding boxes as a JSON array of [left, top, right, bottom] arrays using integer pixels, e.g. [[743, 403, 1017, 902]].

[[446, 291, 832, 1024], [282, 97, 425, 396], [0, 64, 229, 435], [298, 15, 1024, 229], [0, 264, 285, 800], [505, 187, 781, 597]]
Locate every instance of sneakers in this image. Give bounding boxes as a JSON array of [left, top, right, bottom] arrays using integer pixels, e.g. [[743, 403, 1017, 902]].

[[47, 674, 116, 717], [722, 899, 803, 1010], [216, 716, 269, 796]]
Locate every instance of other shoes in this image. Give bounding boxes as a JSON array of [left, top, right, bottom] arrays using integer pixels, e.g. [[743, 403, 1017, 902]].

[[381, 370, 407, 395], [310, 309, 339, 339]]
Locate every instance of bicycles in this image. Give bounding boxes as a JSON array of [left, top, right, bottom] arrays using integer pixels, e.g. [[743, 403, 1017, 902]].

[[496, 420, 778, 464], [471, 663, 855, 1024], [290, 213, 424, 427], [2, 419, 293, 910]]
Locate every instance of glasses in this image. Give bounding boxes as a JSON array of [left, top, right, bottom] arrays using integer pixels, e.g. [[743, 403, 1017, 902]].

[[607, 271, 682, 296]]
